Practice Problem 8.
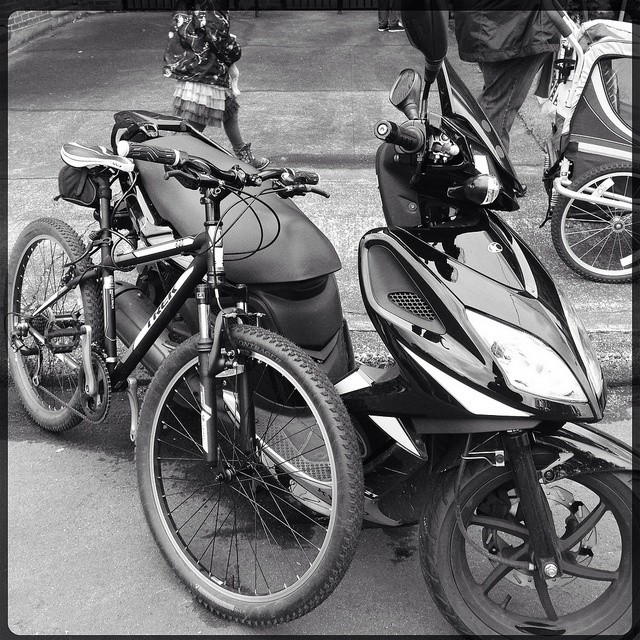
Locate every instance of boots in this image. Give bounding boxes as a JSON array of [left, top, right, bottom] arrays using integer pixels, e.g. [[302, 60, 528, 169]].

[[233, 143, 270, 170]]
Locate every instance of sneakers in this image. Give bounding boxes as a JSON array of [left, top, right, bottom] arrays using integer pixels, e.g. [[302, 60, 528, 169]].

[[378, 23, 389, 32], [388, 24, 405, 32]]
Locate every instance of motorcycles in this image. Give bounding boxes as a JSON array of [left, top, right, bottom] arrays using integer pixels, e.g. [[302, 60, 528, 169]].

[[105, 11, 636, 638]]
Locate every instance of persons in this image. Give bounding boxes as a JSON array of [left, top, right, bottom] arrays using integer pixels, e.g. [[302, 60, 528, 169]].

[[377, 10, 406, 32], [162, 10, 270, 170], [455, 11, 561, 198]]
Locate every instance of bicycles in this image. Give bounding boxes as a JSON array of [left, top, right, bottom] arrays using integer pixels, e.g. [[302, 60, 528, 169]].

[[7, 140, 367, 629]]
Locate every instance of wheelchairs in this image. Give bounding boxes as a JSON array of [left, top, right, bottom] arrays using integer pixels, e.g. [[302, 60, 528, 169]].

[[532, 11, 639, 285]]
